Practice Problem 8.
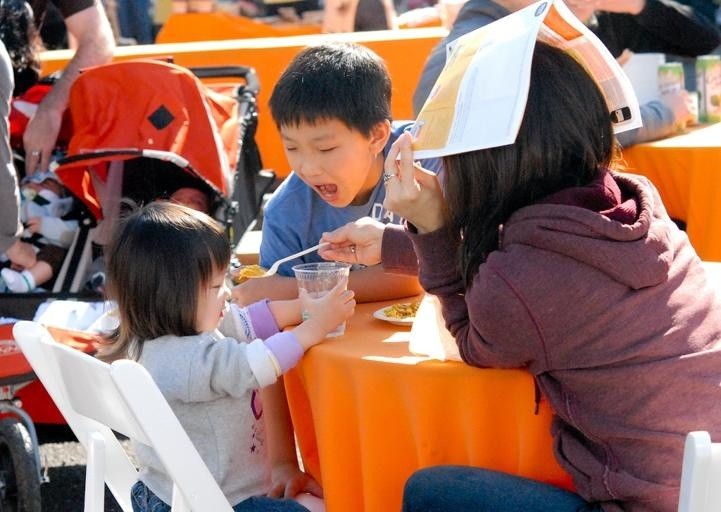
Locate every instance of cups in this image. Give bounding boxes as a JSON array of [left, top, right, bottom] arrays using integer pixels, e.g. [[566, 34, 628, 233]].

[[291, 262, 352, 338]]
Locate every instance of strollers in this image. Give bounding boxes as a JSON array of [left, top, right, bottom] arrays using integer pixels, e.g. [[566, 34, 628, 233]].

[[0, 58, 275, 512]]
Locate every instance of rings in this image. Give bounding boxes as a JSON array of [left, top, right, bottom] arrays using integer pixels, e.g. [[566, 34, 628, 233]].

[[34, 151, 44, 158], [350, 244, 354, 249], [383, 172, 393, 179]]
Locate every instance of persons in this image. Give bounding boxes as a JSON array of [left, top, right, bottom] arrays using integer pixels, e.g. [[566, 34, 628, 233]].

[[0, 37, 37, 271], [84, 199, 357, 511], [413, 1, 694, 149], [230, 41, 445, 500], [0, 180, 81, 294], [316, 39, 720, 512], [601, 1, 720, 62], [91, 187, 208, 286], [1, 0, 115, 176]]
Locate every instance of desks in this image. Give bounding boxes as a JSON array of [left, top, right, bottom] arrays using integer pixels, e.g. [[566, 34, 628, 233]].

[[41, 27, 446, 179], [620, 125, 721, 262]]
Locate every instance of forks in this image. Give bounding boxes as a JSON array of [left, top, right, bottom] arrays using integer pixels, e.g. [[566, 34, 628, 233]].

[[246, 242, 330, 278]]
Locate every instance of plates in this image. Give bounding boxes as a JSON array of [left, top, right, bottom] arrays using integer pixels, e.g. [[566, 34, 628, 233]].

[[373, 302, 422, 327]]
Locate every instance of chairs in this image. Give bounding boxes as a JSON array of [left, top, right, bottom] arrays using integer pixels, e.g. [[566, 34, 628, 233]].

[[678, 431, 721, 512], [12, 321, 236, 512]]
